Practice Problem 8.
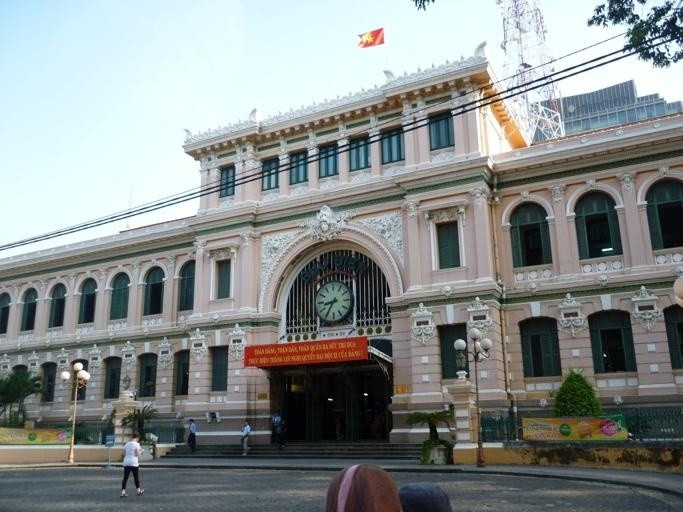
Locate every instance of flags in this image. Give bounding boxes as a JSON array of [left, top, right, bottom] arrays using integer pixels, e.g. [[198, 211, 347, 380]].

[[358, 27, 384, 49]]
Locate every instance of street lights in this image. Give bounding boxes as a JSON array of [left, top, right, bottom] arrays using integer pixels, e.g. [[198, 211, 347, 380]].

[[60, 363, 90, 464], [454, 328, 492, 467]]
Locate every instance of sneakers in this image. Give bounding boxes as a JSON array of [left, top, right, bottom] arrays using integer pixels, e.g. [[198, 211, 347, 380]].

[[135, 488, 144, 495], [120, 493, 129, 497]]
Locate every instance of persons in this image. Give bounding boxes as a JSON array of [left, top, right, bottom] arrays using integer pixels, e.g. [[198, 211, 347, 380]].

[[119, 433, 145, 497], [240, 419, 251, 456], [271, 412, 283, 443], [325, 464, 402, 512], [187, 418, 197, 454], [333, 410, 344, 441], [277, 418, 289, 450]]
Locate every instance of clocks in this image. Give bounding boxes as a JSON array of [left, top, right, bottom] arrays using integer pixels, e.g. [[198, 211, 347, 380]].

[[314, 280, 354, 323]]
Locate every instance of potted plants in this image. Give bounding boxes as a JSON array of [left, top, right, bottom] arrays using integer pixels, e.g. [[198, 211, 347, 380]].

[[120, 403, 160, 462], [407, 409, 454, 465]]
